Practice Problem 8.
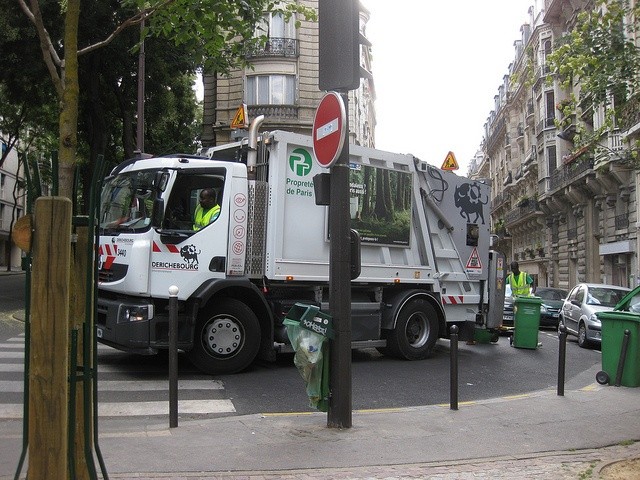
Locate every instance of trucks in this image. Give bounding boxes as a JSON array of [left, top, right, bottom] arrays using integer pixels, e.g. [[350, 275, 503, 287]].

[[94, 116, 494, 374]]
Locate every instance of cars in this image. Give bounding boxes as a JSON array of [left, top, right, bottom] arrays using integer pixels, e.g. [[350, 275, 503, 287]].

[[534, 288, 566, 329], [558, 282, 633, 347], [498, 285, 533, 322]]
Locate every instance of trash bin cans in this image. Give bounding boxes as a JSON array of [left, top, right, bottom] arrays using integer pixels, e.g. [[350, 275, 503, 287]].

[[613, 286, 640, 312], [594, 311, 640, 388], [282, 302, 333, 413], [509, 295, 544, 350], [472, 328, 499, 344]]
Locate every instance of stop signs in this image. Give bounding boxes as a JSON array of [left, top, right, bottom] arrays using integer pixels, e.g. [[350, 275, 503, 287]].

[[313, 92, 347, 168]]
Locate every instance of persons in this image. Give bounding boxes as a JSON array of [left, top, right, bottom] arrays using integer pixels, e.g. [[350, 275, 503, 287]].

[[191, 188, 222, 236], [505, 261, 534, 305]]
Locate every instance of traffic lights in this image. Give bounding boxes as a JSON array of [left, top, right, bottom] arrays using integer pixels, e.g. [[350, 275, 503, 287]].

[[356, 0, 373, 84]]
